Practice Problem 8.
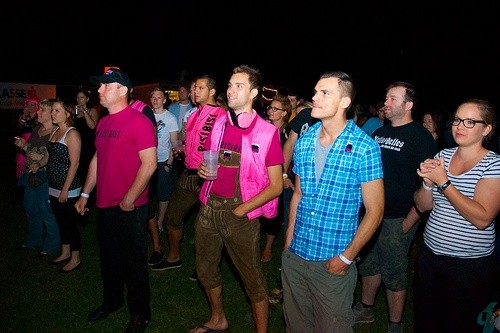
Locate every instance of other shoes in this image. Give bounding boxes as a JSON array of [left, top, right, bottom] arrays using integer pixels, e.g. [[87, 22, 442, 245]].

[[386, 319, 405, 333], [57, 261, 81, 273], [260, 251, 272, 263], [268, 287, 283, 303], [158, 224, 163, 233], [88, 306, 121, 321], [19, 244, 28, 249], [50, 256, 71, 265], [37, 251, 49, 259], [351, 304, 374, 325], [124, 313, 151, 333], [150, 259, 183, 270], [148, 248, 166, 265]]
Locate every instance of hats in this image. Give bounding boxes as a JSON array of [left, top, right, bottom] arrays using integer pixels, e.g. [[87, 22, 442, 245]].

[[95, 68, 131, 89]]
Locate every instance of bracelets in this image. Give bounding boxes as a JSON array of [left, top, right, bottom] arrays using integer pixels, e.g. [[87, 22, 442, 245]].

[[338, 253, 353, 266], [81, 193, 90, 198], [422, 181, 433, 191]]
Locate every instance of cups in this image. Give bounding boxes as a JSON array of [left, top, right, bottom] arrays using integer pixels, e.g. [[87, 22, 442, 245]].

[[203, 150, 219, 180], [172, 140, 184, 160], [74, 105, 80, 115]]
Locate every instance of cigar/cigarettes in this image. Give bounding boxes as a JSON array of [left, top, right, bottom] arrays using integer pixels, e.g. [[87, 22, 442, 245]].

[[28, 167, 34, 174]]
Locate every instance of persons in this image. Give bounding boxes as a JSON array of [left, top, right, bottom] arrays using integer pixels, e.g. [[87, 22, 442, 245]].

[[13, 84, 442, 333], [281, 71, 385, 333], [414, 99, 500, 333], [190, 64, 284, 333], [74, 68, 158, 333]]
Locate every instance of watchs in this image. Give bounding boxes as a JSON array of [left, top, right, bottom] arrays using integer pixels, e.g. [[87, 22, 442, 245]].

[[82, 110, 88, 114], [437, 180, 451, 194]]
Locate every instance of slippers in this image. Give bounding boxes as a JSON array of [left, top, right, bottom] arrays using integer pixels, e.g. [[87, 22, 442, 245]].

[[191, 324, 230, 333]]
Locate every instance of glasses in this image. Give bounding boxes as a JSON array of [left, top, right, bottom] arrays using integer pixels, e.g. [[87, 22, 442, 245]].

[[451, 117, 485, 128], [267, 106, 283, 112]]
